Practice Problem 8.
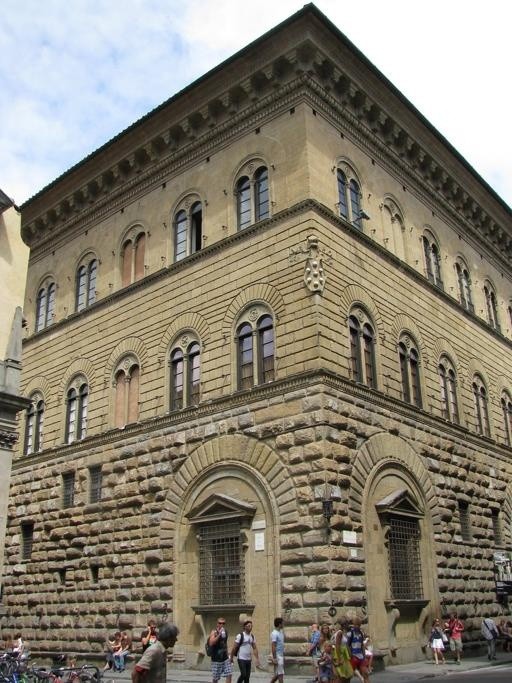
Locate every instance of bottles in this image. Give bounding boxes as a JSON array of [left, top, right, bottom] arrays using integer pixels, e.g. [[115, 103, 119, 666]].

[[221, 627, 226, 637]]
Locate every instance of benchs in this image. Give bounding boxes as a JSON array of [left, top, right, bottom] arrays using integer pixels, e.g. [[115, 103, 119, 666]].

[[28, 651, 174, 671], [266, 648, 390, 676], [441, 637, 507, 657]]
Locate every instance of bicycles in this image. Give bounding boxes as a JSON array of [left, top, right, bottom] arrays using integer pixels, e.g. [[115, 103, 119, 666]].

[[1, 643, 115, 682]]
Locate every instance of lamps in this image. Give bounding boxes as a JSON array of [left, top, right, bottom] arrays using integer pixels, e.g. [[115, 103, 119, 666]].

[[322, 497, 333, 535]]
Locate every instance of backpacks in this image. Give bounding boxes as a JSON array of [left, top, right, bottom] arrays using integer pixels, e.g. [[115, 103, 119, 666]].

[[204, 628, 219, 657]]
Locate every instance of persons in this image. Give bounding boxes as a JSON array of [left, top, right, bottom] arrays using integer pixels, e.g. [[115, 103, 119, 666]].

[[505, 621, 512, 635], [131, 624, 179, 683], [306, 616, 373, 683], [205, 617, 232, 683], [269, 618, 284, 683], [481, 613, 499, 660], [230, 621, 260, 683], [141, 620, 159, 652], [444, 614, 464, 665], [425, 618, 448, 665], [103, 631, 132, 673], [496, 620, 512, 652]]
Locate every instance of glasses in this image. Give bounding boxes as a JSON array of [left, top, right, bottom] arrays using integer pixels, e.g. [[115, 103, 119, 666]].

[[218, 622, 226, 625]]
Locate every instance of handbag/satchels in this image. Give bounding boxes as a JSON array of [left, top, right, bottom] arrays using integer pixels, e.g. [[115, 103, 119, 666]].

[[489, 629, 498, 639]]
